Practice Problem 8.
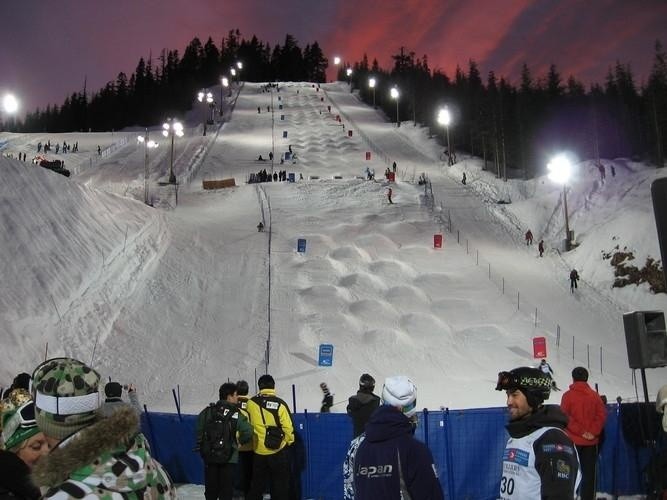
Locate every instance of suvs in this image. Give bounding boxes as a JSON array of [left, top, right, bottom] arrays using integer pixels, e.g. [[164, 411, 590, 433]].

[[41, 159, 72, 178]]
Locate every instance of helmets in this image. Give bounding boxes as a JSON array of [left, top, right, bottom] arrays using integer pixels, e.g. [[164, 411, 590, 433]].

[[510, 367, 549, 406]]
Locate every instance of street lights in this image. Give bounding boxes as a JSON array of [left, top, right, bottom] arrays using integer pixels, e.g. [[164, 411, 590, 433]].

[[435, 102, 452, 166], [218, 61, 245, 116], [135, 127, 160, 205], [332, 56, 378, 112], [196, 86, 214, 137], [161, 116, 185, 185], [389, 84, 400, 128], [546, 151, 579, 253]]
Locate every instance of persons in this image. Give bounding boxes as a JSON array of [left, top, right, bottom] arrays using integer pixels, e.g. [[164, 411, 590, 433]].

[[526, 229, 534, 247], [343, 376, 446, 500], [347, 373, 381, 441], [461, 172, 467, 186], [561, 367, 610, 500], [228, 380, 257, 500], [569, 269, 580, 289], [192, 382, 255, 500], [1, 356, 178, 500], [319, 381, 335, 412], [247, 374, 297, 500], [36, 139, 79, 155], [538, 240, 545, 257], [495, 368, 586, 499], [255, 169, 304, 183], [387, 188, 394, 205], [539, 359, 557, 389], [365, 161, 395, 181]]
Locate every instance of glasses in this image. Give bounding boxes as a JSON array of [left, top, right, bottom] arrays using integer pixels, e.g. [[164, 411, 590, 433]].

[[3, 399, 36, 445], [495, 372, 548, 390]]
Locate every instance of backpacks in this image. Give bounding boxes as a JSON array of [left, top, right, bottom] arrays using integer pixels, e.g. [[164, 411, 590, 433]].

[[200, 406, 238, 464]]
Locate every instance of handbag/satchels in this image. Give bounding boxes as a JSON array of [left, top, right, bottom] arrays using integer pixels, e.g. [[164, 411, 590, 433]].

[[264, 426, 282, 449]]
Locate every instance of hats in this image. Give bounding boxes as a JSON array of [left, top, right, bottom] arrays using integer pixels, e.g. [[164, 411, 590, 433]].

[[3, 405, 41, 449], [359, 374, 375, 392], [381, 377, 417, 418], [33, 360, 101, 440], [105, 383, 122, 398]]
